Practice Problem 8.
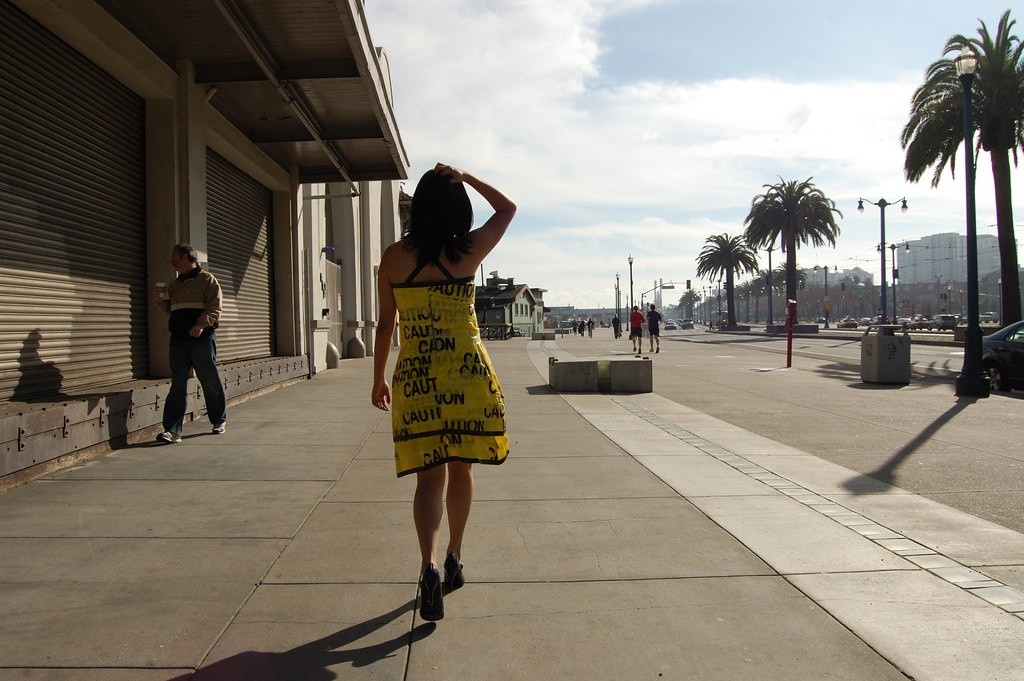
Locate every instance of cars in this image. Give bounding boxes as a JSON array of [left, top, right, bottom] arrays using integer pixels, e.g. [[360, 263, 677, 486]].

[[929, 314, 957, 331], [860, 318, 873, 326], [664, 318, 694, 330], [815, 317, 825, 323], [906, 317, 932, 330], [979, 311, 999, 323], [837, 318, 858, 328], [897, 318, 911, 330], [982, 320, 1024, 392], [874, 316, 890, 329], [562, 320, 588, 328]]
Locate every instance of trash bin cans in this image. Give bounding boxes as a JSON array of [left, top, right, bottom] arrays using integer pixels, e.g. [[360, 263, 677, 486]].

[[860, 324, 912, 384]]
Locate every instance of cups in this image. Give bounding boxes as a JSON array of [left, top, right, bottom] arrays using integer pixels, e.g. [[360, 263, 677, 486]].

[[155, 282, 166, 297]]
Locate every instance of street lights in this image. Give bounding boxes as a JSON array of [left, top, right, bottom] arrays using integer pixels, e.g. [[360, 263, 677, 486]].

[[960, 289, 962, 325], [754, 246, 782, 325], [857, 196, 908, 336], [814, 265, 838, 328], [948, 285, 951, 314], [614, 254, 633, 331], [694, 279, 722, 324], [998, 279, 1002, 325], [935, 274, 944, 307]]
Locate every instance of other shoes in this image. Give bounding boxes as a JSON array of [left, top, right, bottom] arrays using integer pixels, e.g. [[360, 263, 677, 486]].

[[649, 346, 660, 353], [632, 347, 641, 354]]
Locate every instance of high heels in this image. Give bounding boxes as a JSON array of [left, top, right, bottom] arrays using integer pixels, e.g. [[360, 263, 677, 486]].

[[444, 553, 465, 594], [419, 563, 444, 622]]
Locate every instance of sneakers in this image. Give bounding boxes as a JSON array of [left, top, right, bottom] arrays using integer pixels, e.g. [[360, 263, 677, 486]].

[[212, 421, 227, 434], [156, 431, 183, 443]]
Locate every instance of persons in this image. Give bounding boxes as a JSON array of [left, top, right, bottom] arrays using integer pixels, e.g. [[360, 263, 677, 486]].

[[578, 321, 585, 336], [630, 306, 646, 354], [586, 319, 594, 338], [709, 320, 714, 330], [612, 314, 620, 339], [371, 163, 517, 622], [154, 241, 227, 443], [647, 304, 662, 353]]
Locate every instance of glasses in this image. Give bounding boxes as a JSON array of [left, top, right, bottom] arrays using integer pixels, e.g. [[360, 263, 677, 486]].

[[170, 255, 180, 260]]
